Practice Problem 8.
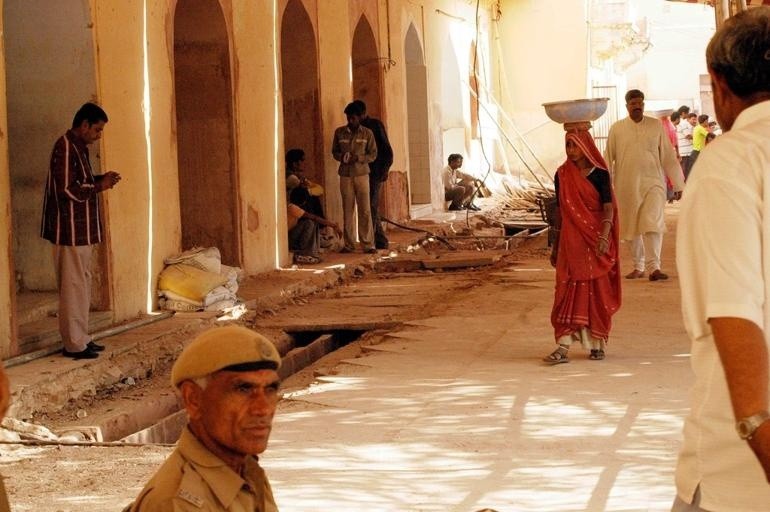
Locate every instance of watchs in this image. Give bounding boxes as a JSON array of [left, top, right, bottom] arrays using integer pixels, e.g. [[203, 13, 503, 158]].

[[736, 409, 769, 438]]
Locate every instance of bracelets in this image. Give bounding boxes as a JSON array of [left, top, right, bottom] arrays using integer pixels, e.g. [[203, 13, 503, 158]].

[[597, 234, 609, 244], [602, 219, 613, 227]]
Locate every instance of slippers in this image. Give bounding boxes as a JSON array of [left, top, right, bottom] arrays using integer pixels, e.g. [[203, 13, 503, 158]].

[[589, 349, 605, 361], [542, 349, 571, 362]]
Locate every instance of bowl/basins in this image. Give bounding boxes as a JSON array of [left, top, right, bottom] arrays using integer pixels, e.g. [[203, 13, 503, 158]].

[[542, 98, 609, 122], [654, 109, 673, 117]]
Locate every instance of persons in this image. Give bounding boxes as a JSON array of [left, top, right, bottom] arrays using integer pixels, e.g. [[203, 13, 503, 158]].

[[689, 115, 716, 175], [355, 99, 394, 248], [543, 130, 621, 362], [286, 182, 342, 264], [674, 8, 770, 511], [687, 113, 697, 126], [41, 103, 121, 359], [285, 149, 333, 247], [603, 90, 685, 281], [678, 105, 693, 182], [669, 111, 680, 130], [117, 325, 282, 512], [333, 103, 377, 254], [708, 117, 717, 142], [657, 118, 681, 203], [443, 154, 480, 210]]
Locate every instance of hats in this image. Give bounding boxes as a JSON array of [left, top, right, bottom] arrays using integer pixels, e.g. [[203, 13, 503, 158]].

[[171, 324, 282, 388]]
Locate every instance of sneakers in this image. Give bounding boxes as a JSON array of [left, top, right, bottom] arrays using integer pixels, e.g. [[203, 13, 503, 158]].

[[62, 347, 99, 359], [367, 248, 378, 253], [294, 255, 319, 264], [448, 205, 466, 210], [626, 270, 645, 279], [309, 254, 324, 261], [340, 247, 352, 253], [464, 204, 480, 211], [87, 341, 105, 351], [649, 272, 668, 281]]
